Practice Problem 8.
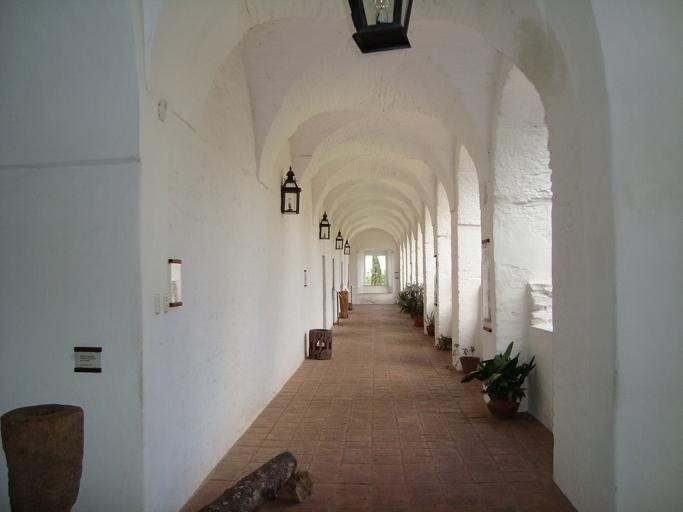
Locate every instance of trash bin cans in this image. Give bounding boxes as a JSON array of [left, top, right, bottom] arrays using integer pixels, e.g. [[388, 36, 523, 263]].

[[310, 329, 332, 360]]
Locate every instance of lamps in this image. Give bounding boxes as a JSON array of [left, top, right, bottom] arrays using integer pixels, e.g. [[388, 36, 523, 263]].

[[281, 166, 300, 214], [319, 211, 351, 256], [347, 0, 412, 54]]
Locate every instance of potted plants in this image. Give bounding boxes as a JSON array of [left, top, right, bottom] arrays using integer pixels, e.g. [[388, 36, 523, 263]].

[[461, 340, 537, 421], [397, 280, 436, 337]]
